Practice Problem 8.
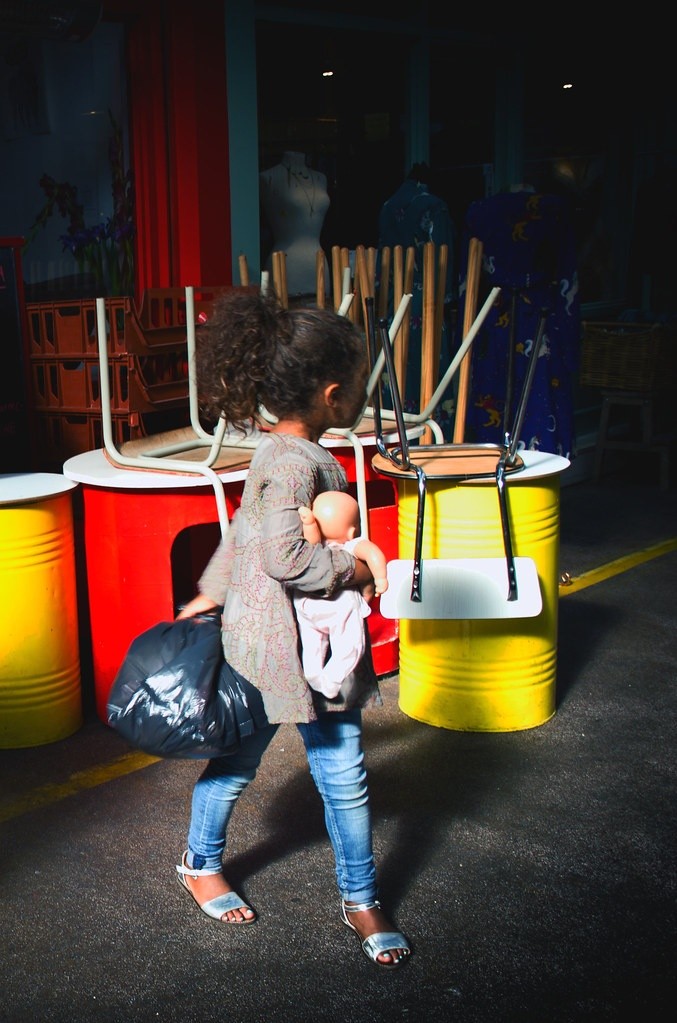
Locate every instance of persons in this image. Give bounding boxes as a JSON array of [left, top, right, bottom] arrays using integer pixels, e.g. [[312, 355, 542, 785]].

[[294, 491, 387, 700], [258, 151, 330, 299], [379, 161, 581, 463], [176, 286, 411, 966]]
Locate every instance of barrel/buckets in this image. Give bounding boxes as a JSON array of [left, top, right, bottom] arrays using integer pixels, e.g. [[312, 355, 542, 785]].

[[0, 493, 80, 748], [398, 452, 557, 732]]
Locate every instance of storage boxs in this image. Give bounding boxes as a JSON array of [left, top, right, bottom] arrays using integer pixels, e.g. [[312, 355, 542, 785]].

[[29, 284, 205, 460]]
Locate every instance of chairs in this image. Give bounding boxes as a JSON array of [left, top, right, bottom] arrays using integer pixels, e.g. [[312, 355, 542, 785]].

[[93, 233, 572, 621]]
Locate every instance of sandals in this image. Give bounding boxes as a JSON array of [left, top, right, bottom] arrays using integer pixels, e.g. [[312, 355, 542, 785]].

[[340, 898, 412, 971], [176, 851, 257, 926]]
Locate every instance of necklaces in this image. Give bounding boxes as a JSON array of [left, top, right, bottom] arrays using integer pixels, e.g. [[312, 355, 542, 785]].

[[282, 162, 315, 213]]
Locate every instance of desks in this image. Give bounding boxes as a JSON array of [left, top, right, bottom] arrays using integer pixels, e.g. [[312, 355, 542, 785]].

[[595, 389, 675, 489]]
